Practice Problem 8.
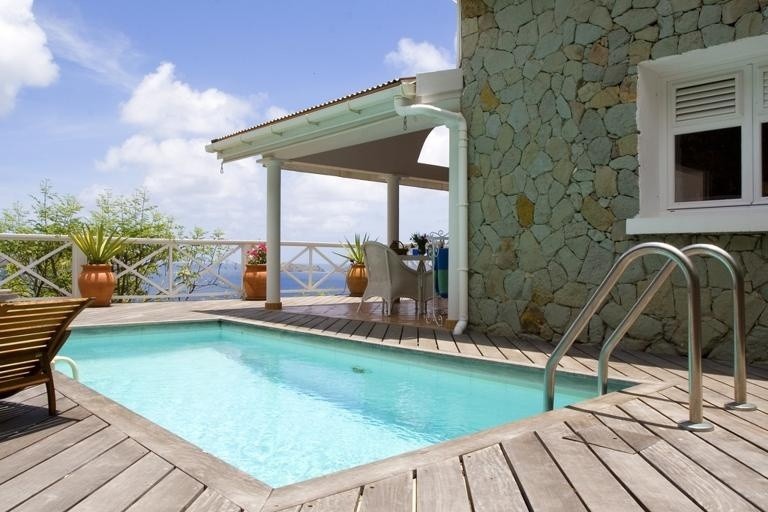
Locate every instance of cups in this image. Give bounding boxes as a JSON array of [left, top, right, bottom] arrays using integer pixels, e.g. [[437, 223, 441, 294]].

[[413, 248, 418, 256]]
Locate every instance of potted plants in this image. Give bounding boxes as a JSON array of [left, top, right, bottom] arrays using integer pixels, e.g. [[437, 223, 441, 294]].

[[334, 230, 379, 298], [411, 234, 429, 253], [69, 220, 149, 308]]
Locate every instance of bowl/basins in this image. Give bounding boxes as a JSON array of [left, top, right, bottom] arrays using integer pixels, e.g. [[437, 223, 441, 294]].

[[395, 248, 409, 254]]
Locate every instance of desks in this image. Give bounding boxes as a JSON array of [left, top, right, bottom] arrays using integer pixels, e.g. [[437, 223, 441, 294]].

[[395, 253, 433, 262]]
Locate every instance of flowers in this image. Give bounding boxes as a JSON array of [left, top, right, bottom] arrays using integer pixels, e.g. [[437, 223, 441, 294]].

[[247, 242, 267, 263]]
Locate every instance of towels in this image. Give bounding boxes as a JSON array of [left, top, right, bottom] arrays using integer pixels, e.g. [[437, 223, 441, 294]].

[[432, 246, 448, 297]]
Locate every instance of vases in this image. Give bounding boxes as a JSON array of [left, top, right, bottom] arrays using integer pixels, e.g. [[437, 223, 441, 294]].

[[241, 263, 265, 300]]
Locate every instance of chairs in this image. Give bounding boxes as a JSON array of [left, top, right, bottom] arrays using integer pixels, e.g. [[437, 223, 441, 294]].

[[0, 293, 88, 420], [356, 240, 421, 316]]
